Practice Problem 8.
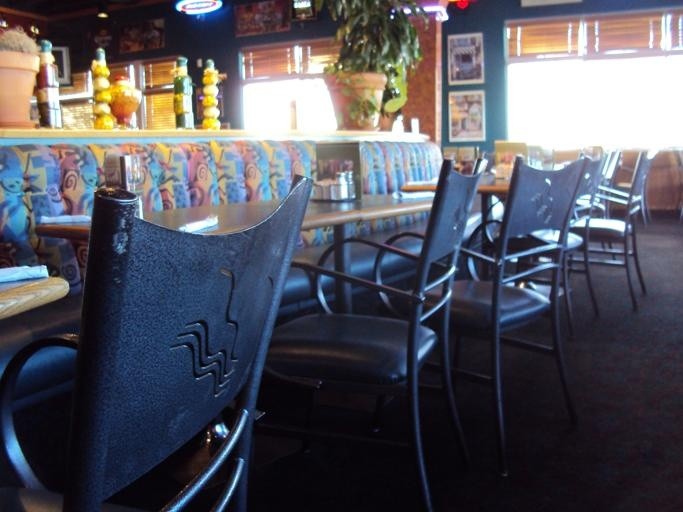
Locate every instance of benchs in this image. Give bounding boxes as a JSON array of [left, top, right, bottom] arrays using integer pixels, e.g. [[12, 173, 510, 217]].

[[0, 139, 502, 354]]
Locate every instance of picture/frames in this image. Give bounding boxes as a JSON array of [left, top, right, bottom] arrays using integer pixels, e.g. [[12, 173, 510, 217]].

[[448, 90, 486, 143], [447, 33, 485, 85]]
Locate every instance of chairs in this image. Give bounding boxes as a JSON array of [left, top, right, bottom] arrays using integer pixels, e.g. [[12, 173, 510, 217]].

[[261, 157, 487, 512], [0, 174, 314, 512]]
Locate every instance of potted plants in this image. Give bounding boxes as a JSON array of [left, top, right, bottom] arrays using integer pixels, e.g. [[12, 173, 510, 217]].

[[314, 0, 429, 130], [0, 30, 40, 127]]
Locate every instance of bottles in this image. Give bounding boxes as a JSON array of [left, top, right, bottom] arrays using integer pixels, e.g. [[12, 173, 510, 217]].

[[542, 156, 554, 170], [330, 173, 348, 199], [503, 155, 514, 181], [344, 170, 355, 196], [174, 58, 193, 127], [36, 41, 61, 127], [531, 152, 544, 170]]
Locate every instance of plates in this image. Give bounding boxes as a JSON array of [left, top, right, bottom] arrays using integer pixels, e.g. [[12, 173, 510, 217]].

[[310, 197, 357, 204]]
